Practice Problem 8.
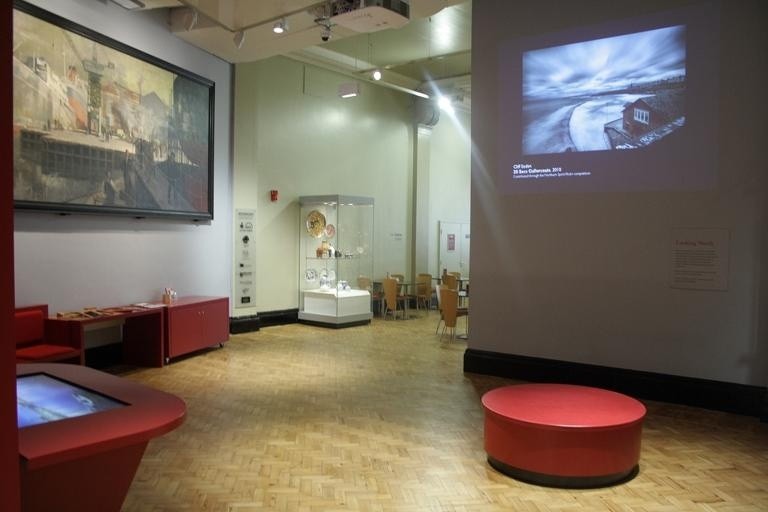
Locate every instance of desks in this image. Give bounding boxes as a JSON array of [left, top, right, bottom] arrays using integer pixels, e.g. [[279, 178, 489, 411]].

[[55, 300, 166, 371]]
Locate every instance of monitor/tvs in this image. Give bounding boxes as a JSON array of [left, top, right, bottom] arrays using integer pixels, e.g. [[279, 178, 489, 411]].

[[17, 373, 131, 429]]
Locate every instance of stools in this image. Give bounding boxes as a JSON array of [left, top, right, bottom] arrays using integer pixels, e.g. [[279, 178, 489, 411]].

[[477, 381, 647, 493]]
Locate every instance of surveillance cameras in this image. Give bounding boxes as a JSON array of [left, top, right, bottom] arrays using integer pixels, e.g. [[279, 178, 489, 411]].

[[321, 30, 331, 41]]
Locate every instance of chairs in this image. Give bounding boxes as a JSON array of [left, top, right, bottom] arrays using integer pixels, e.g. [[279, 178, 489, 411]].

[[16, 301, 78, 364], [358, 262, 468, 344]]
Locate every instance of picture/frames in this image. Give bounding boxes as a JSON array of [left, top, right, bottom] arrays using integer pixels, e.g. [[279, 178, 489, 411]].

[[11, 2, 218, 225]]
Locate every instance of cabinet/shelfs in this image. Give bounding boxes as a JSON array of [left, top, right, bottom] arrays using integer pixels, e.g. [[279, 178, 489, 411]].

[[136, 291, 232, 365]]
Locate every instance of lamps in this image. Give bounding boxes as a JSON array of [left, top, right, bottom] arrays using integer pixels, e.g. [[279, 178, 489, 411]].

[[367, 28, 385, 83], [229, 11, 291, 49]]
